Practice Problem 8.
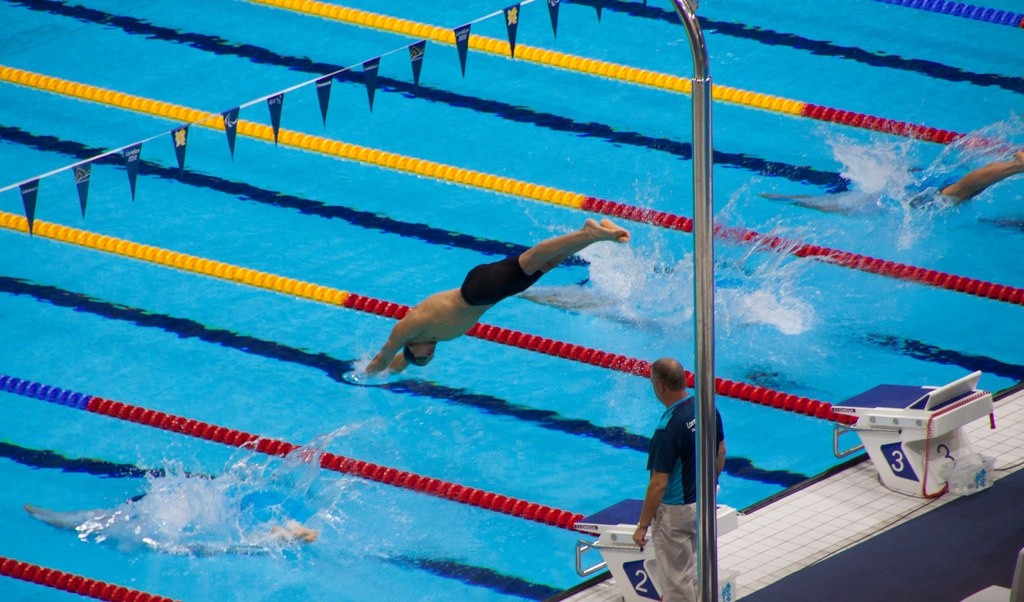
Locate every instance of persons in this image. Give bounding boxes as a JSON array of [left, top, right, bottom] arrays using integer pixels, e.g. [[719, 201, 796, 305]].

[[633, 358, 726, 602], [941, 148, 1024, 201], [365, 217, 631, 376], [270, 520, 318, 543]]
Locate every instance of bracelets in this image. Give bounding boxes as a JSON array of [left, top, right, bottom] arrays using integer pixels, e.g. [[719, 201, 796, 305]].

[[638, 522, 650, 530]]
